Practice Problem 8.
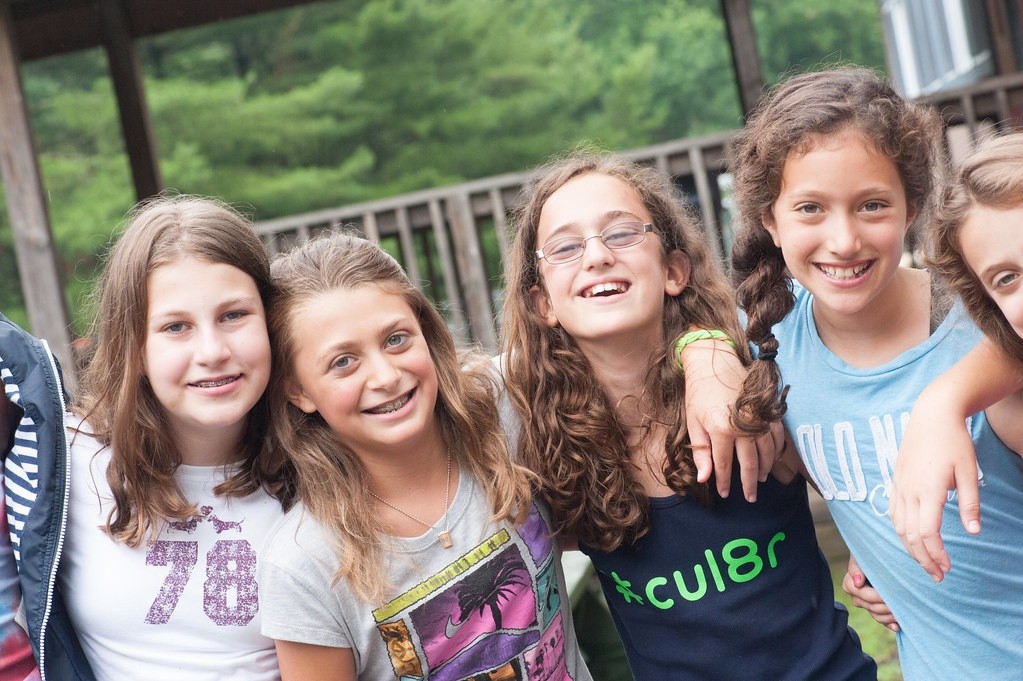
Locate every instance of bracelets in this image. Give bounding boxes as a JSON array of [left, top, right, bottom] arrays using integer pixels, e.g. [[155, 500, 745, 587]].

[[673, 330, 741, 365]]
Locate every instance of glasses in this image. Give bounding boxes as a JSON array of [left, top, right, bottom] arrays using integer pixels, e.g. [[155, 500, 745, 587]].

[[534, 220, 673, 265]]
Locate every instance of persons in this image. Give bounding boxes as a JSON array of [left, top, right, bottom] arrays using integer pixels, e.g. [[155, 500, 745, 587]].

[[889, 123, 1023, 573], [46, 192, 318, 679], [261, 235, 793, 679], [510, 151, 877, 678], [1, 313, 93, 679], [724, 63, 1023, 681]]
